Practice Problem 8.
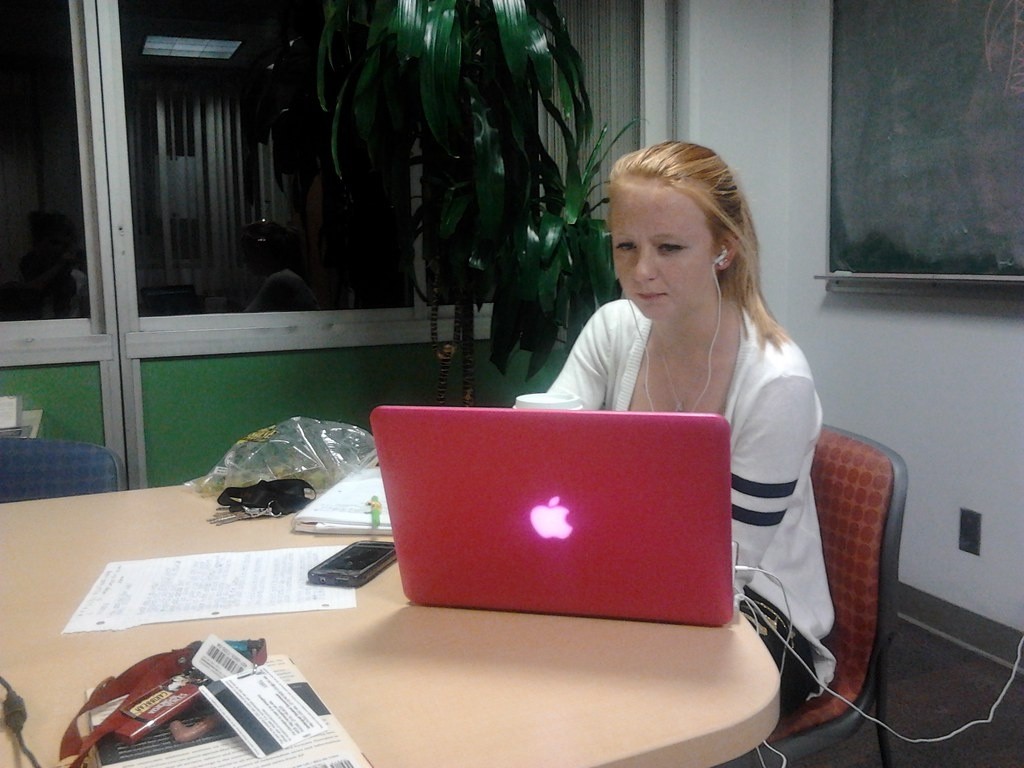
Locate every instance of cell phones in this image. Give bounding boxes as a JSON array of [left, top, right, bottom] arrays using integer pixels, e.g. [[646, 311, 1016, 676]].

[[308, 540, 397, 587]]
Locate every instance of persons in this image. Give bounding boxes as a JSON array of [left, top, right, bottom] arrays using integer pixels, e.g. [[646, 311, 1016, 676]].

[[20, 249, 91, 320], [549, 141, 837, 718], [237, 221, 320, 313]]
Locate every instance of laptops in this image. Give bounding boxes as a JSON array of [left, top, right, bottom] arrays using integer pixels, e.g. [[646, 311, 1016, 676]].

[[369, 405, 734, 626]]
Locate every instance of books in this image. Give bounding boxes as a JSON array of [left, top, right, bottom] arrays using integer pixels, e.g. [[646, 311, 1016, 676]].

[[292, 466, 392, 536], [77, 655, 374, 768]]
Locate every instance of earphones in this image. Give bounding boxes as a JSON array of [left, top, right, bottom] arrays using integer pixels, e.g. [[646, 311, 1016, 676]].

[[714, 245, 726, 264]]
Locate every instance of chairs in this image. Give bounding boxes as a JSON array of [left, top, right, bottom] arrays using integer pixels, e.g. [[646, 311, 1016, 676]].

[[719, 423, 909, 768]]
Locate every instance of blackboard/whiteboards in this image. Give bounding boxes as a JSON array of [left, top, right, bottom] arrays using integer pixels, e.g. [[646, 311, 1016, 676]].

[[818, 0, 1024, 298]]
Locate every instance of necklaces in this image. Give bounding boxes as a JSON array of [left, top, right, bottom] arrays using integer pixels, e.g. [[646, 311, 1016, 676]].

[[657, 344, 688, 413]]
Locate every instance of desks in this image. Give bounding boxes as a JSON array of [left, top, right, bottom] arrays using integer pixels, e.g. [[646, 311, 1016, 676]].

[[0, 474, 779, 768]]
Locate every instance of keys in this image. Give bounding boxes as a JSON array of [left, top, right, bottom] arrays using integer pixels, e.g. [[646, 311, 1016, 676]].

[[207, 501, 282, 526]]
